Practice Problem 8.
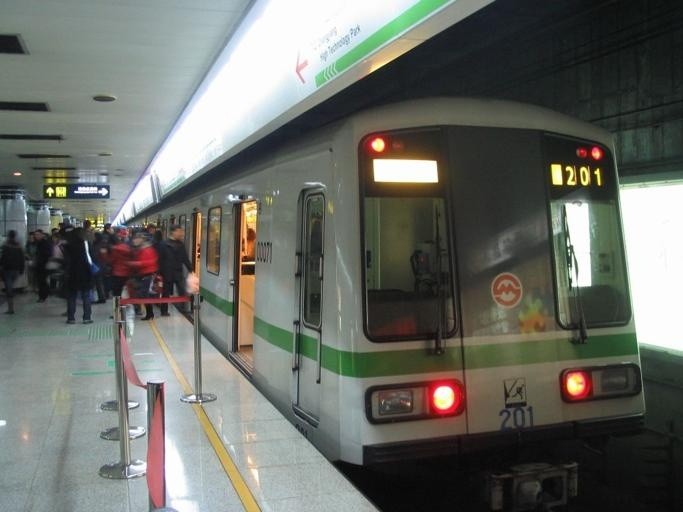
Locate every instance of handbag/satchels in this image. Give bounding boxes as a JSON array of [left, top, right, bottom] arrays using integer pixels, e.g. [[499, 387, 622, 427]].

[[185, 271, 200, 294], [148, 272, 164, 294]]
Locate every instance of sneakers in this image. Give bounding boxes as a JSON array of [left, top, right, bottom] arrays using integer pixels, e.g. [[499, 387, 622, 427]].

[[67, 319, 75, 324], [161, 312, 170, 316], [83, 319, 94, 323]]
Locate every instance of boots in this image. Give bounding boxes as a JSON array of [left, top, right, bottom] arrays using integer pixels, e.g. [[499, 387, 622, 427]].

[[141, 304, 154, 320]]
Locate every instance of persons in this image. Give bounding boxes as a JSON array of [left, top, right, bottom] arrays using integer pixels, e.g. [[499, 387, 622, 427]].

[[0, 220, 255, 326]]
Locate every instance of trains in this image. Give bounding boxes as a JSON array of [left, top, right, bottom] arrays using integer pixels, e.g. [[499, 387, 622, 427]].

[[127, 94, 647, 473]]
